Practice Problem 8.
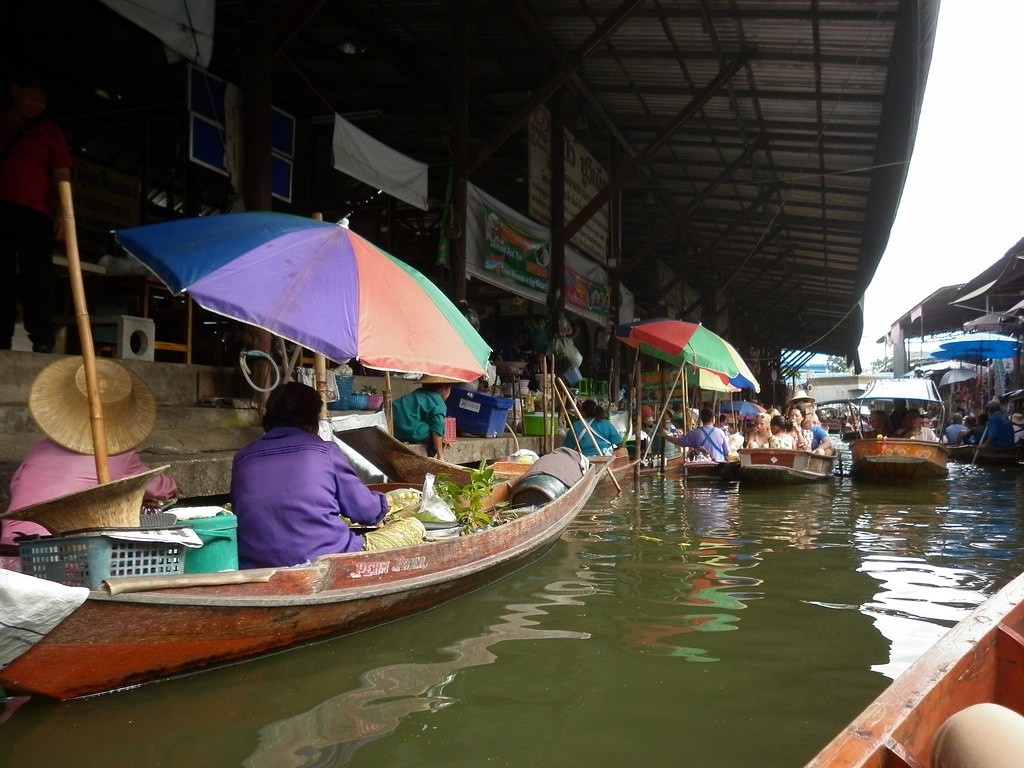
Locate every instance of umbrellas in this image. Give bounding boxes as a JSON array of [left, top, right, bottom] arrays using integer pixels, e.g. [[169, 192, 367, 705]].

[[685, 367, 743, 420], [902, 348, 998, 398], [719, 398, 768, 420], [610, 318, 763, 464], [940, 330, 1023, 403], [108, 213, 492, 390]]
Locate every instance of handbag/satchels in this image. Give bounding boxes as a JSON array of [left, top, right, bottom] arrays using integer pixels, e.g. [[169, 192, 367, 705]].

[[530, 312, 583, 369]]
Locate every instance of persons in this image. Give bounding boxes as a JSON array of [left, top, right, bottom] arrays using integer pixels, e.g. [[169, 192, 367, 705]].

[[659, 414, 745, 466], [0, 71, 75, 354], [1, 349, 179, 576], [843, 414, 866, 431], [869, 396, 939, 444], [742, 388, 835, 456], [392, 374, 462, 465], [944, 398, 1024, 447], [561, 400, 623, 460], [225, 381, 428, 571]]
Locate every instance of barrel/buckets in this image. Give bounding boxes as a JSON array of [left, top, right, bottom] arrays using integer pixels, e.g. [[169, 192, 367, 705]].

[[172, 509, 239, 576], [510, 447, 582, 510]]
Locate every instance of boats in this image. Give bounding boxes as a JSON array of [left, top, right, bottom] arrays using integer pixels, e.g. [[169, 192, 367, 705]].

[[0, 329, 1020, 707]]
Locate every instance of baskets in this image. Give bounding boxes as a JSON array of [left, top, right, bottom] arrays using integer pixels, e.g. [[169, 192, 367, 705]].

[[13, 536, 186, 591], [351, 393, 369, 410], [365, 393, 384, 411], [444, 418, 458, 442], [325, 376, 356, 411]]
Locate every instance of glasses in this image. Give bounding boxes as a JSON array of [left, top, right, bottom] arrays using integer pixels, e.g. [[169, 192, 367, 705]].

[[755, 414, 766, 420]]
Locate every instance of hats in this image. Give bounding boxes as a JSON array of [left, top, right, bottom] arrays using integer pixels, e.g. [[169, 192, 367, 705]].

[[1, 463, 171, 550], [1008, 412, 1024, 424], [786, 391, 815, 407], [414, 374, 466, 384], [28, 354, 158, 456]]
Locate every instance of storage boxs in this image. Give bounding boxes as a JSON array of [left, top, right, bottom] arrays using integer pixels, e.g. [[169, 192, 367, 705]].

[[445, 386, 515, 437], [523, 411, 559, 436]]
